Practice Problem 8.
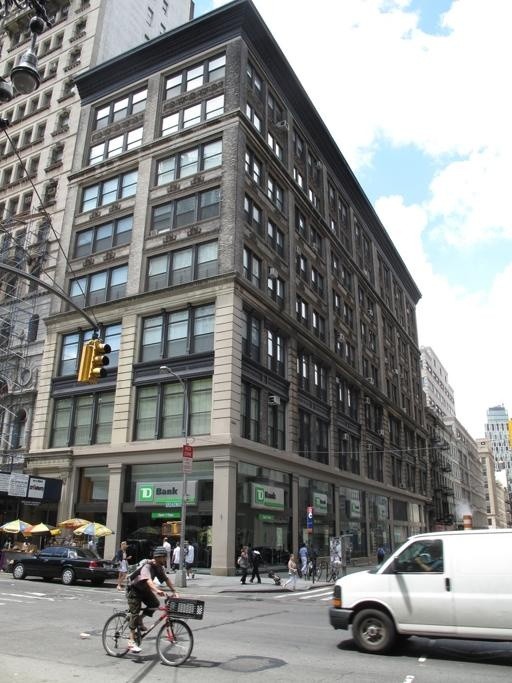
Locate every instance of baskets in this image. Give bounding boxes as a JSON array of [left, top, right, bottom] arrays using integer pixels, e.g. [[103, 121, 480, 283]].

[[166, 598, 204, 620]]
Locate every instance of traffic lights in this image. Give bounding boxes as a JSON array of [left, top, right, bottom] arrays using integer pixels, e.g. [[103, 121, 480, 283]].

[[78, 339, 111, 384]]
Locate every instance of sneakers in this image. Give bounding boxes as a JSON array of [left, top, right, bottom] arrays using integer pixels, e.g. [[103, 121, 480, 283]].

[[128, 641, 141, 652], [134, 618, 147, 631]]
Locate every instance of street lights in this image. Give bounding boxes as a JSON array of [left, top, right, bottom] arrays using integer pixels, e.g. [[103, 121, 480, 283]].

[[160, 364, 191, 588]]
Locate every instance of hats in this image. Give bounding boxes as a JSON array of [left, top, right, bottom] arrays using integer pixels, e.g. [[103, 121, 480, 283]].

[[154, 547, 167, 557]]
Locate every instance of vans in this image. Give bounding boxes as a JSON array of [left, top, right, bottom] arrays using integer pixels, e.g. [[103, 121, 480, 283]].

[[329, 528, 512, 654]]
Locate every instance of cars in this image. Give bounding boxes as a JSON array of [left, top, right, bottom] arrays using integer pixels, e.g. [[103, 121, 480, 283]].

[[8, 546, 120, 585]]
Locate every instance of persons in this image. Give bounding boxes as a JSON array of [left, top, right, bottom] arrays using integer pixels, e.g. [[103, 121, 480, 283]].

[[162, 536, 196, 580], [12, 537, 37, 553], [416, 544, 443, 571], [126, 545, 177, 653], [281, 541, 318, 591], [238, 545, 263, 584], [377, 543, 385, 563], [116, 540, 131, 590], [88, 537, 101, 562], [63, 535, 72, 546]]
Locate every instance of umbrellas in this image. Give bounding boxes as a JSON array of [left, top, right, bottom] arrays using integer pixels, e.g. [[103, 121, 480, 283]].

[[58, 516, 91, 529], [0, 518, 33, 533], [76, 521, 114, 538], [24, 522, 62, 536]]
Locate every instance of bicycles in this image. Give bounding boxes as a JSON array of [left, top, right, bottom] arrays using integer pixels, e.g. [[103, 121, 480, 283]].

[[312, 561, 339, 582], [102, 592, 204, 666]]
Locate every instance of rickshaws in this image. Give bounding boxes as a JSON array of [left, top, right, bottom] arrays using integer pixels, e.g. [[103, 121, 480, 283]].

[[263, 561, 281, 585]]
